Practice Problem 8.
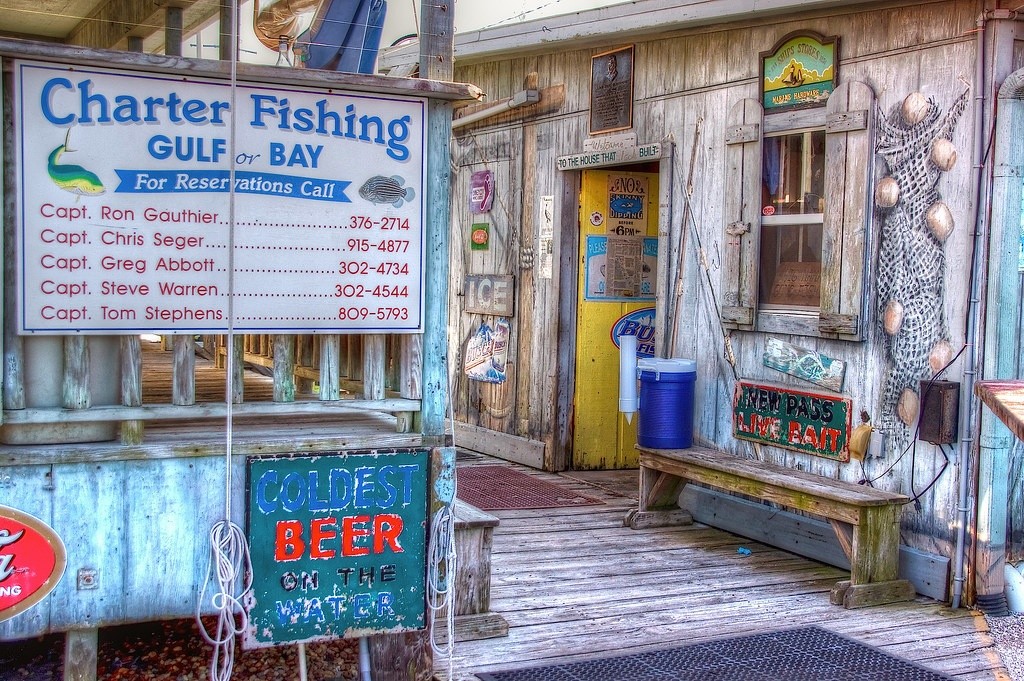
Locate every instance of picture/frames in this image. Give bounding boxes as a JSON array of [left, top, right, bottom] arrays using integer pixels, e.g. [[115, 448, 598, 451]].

[[587, 44, 633, 135], [757, 30, 841, 116]]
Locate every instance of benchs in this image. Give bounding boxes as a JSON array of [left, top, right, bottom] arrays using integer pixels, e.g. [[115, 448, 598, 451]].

[[428, 497, 510, 646], [633, 442, 916, 609]]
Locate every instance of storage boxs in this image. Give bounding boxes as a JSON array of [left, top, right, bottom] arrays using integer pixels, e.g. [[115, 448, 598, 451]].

[[634, 357, 698, 449]]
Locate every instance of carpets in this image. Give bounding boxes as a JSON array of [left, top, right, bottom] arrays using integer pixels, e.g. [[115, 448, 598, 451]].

[[455, 462, 608, 510], [473, 625, 965, 681]]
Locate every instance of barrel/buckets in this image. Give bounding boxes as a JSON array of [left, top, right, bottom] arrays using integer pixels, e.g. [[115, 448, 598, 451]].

[[635, 358, 697, 449]]
